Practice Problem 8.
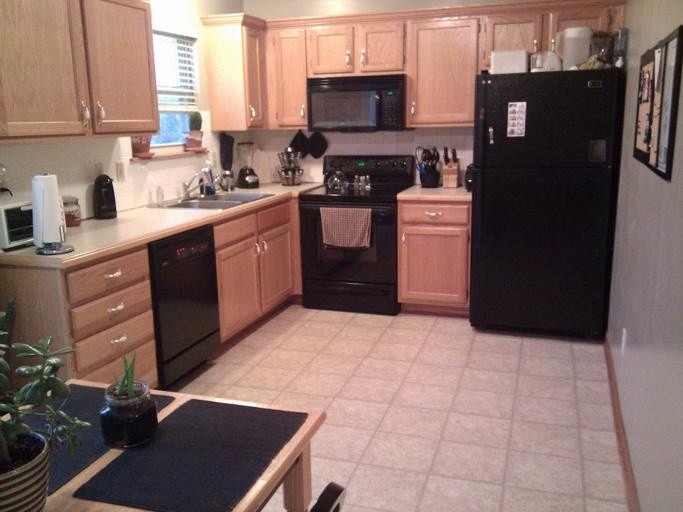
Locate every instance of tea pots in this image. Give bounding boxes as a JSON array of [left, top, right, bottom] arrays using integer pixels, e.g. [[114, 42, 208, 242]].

[[323, 162, 349, 190]]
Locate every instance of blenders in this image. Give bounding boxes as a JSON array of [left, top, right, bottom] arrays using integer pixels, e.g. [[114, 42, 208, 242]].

[[236, 141, 259, 189]]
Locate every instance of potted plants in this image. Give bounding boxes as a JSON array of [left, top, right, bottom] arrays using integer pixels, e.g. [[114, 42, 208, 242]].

[[186, 110, 205, 148], [0, 293, 91, 512], [96, 352, 159, 446]]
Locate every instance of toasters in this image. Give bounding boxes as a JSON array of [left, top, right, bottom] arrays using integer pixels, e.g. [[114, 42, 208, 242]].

[[0, 194, 66, 251]]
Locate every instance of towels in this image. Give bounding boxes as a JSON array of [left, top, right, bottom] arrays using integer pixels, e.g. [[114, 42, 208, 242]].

[[317, 207, 374, 250]]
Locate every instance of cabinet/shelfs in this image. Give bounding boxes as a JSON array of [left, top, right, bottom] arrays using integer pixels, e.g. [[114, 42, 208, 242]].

[[405, 6, 481, 130], [264, 18, 311, 132], [212, 191, 294, 347], [396, 184, 473, 319], [306, 10, 407, 76], [199, 11, 269, 133], [480, 1, 627, 75], [1, 2, 159, 141], [63, 249, 158, 388]]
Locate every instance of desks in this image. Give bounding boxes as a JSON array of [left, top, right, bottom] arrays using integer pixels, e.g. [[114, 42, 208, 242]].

[[1, 374, 329, 512]]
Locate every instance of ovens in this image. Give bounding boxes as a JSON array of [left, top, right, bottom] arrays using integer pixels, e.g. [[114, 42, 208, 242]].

[[298, 205, 400, 317]]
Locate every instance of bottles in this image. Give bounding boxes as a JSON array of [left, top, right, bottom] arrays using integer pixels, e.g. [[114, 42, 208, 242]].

[[354, 174, 370, 191], [62, 195, 82, 228]]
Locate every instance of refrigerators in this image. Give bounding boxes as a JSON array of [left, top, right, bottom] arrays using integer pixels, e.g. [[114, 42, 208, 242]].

[[470, 72, 627, 341]]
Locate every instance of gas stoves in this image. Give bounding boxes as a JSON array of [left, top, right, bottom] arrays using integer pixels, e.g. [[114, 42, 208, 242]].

[[298, 184, 411, 205]]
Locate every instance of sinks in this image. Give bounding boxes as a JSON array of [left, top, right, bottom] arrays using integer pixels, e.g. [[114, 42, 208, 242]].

[[146, 199, 242, 213], [194, 193, 276, 203]]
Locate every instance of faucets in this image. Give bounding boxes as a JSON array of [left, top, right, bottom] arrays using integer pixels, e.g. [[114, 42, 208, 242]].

[[181, 174, 208, 202]]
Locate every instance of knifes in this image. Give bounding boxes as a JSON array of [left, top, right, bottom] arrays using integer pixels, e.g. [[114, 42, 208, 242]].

[[442, 146, 457, 165]]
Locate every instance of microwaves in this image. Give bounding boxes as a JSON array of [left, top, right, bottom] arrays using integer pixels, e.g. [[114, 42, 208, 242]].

[[305, 75, 407, 131]]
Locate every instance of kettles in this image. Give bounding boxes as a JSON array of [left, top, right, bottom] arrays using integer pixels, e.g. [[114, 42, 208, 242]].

[[91, 175, 117, 220]]
